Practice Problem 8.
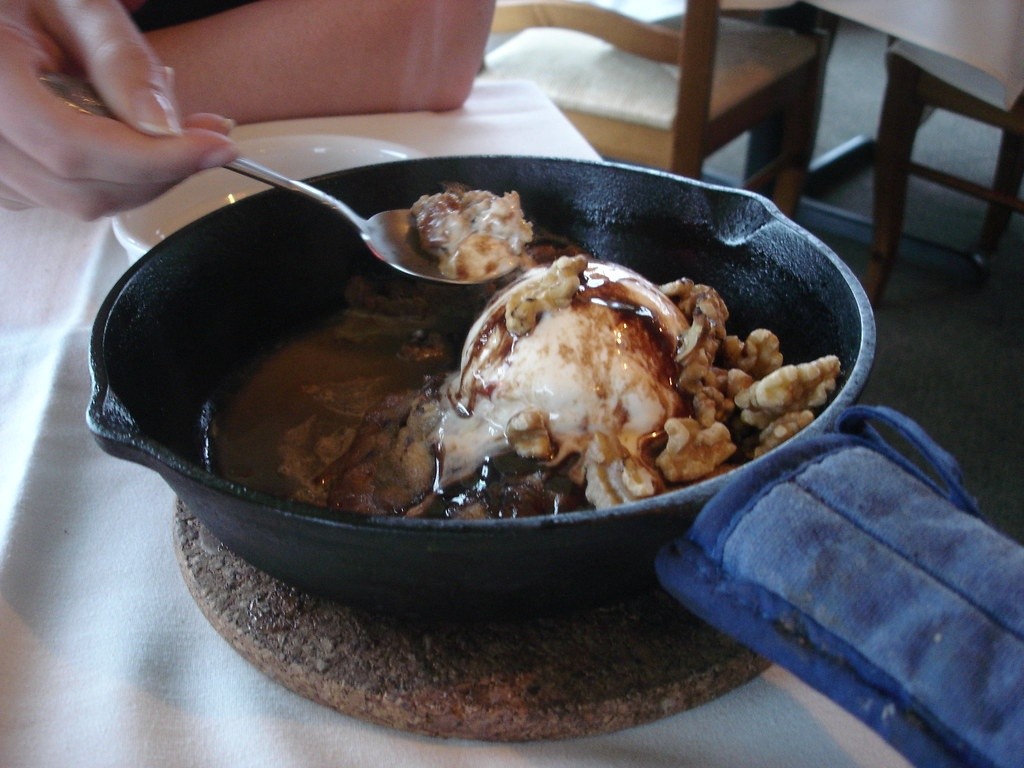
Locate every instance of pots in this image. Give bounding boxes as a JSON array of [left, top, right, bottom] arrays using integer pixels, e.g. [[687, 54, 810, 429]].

[[86, 156, 879, 636]]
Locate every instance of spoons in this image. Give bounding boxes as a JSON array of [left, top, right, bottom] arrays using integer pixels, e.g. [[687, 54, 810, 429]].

[[40, 73, 518, 284]]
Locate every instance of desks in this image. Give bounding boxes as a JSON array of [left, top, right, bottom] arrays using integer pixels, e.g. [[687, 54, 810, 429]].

[[0, 74, 916, 768]]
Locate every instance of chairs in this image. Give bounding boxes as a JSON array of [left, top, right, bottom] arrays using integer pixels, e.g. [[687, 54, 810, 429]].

[[478, 0, 1024, 302]]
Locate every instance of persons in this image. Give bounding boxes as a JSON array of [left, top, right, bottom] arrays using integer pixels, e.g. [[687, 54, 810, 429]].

[[0, 0, 497, 223]]
